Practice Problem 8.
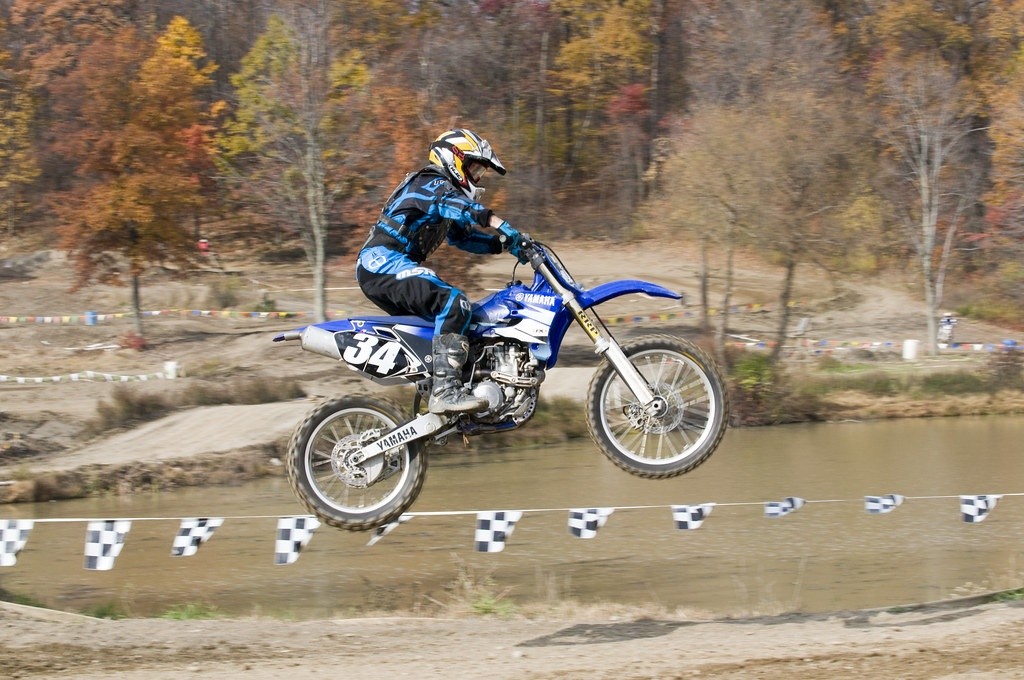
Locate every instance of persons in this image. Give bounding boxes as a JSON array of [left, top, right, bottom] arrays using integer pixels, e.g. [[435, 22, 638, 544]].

[[939, 313, 957, 326], [357, 128, 530, 413]]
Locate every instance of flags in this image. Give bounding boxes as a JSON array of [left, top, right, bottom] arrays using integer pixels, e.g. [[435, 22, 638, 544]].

[[567, 509, 614, 539], [83, 519, 130, 571], [170, 519, 224, 556], [0, 519, 35, 566], [864, 494, 904, 515], [763, 497, 806, 517], [274, 517, 321, 565], [959, 494, 1004, 524], [671, 503, 713, 529], [366, 516, 415, 545], [472, 512, 523, 553]]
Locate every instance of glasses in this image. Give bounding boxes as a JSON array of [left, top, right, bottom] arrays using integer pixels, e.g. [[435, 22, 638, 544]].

[[465, 160, 487, 184]]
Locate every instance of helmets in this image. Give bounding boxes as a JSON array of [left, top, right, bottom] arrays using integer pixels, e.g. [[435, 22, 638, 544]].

[[429, 128, 506, 203]]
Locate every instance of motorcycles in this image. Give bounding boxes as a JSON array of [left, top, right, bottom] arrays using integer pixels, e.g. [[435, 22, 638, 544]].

[[271, 232, 731, 531]]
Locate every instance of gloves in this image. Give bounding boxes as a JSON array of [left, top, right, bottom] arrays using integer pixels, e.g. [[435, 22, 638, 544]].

[[496, 221, 523, 252], [504, 244, 530, 265]]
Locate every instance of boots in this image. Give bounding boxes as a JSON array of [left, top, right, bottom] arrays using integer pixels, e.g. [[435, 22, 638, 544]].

[[428, 334, 489, 415]]
